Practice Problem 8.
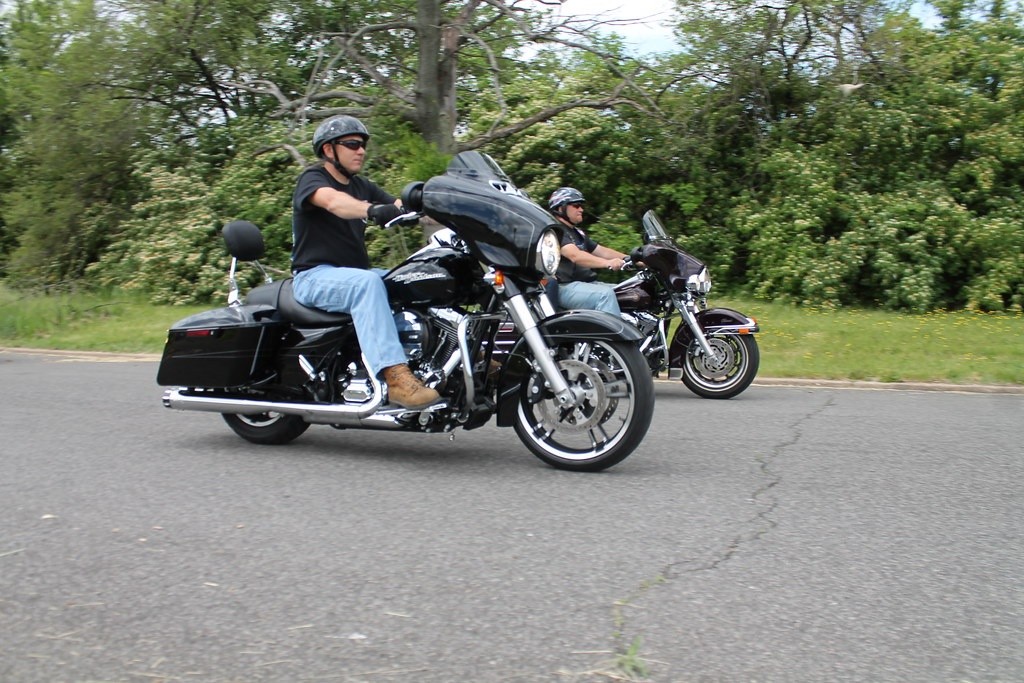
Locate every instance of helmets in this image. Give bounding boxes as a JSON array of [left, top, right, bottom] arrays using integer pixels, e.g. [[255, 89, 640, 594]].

[[312, 114, 370, 157], [549, 188, 586, 210]]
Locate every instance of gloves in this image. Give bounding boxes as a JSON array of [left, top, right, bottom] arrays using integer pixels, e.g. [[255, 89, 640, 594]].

[[368, 205, 404, 230], [398, 207, 419, 228]]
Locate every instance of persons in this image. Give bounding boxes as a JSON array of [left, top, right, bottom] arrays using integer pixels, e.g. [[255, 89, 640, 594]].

[[550, 187, 646, 373], [292, 115, 443, 410]]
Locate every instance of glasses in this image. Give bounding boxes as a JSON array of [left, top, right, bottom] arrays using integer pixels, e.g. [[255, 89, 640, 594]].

[[329, 140, 366, 150], [567, 202, 582, 209]]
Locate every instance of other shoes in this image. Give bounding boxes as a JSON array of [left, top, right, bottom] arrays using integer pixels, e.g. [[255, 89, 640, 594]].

[[589, 355, 623, 373]]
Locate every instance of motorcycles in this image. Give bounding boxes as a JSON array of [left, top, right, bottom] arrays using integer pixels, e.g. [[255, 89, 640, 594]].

[[416, 209, 764, 401], [154, 151, 654, 471]]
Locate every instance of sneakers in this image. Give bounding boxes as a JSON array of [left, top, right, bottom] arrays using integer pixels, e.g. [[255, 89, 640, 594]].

[[383, 364, 442, 410]]
